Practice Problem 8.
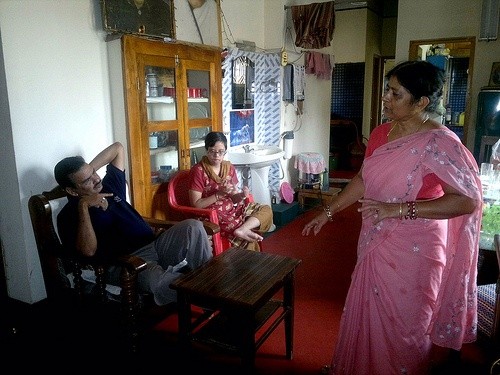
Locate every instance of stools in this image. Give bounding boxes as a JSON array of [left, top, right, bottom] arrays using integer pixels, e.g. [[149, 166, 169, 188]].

[[299, 187, 341, 210]]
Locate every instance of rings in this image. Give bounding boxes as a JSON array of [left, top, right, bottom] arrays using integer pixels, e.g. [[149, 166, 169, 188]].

[[101, 200, 104, 203], [375, 209, 379, 214], [102, 197, 106, 200]]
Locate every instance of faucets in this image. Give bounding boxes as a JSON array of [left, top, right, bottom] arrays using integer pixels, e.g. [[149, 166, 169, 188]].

[[246, 145, 254, 153]]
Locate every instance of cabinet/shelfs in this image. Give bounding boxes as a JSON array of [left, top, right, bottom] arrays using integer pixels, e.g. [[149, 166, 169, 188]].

[[109, 36, 222, 220]]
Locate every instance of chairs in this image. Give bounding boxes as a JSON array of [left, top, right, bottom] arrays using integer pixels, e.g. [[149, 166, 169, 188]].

[[479, 135, 499, 167], [167, 169, 255, 255], [475, 235, 500, 375], [29, 183, 218, 362]]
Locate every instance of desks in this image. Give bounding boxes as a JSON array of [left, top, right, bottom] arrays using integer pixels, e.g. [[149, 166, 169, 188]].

[[168, 249, 301, 375], [478, 167, 500, 253]]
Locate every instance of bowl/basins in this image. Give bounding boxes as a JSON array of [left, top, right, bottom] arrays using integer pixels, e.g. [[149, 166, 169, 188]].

[[280, 182, 293, 203]]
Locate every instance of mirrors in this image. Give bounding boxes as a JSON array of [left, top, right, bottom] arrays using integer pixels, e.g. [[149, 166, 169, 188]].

[[408, 36, 477, 149], [232, 56, 257, 110]]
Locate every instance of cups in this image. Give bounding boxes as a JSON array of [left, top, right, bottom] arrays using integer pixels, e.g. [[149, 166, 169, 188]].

[[186, 87, 207, 98], [164, 87, 175, 97], [481, 163, 493, 178]]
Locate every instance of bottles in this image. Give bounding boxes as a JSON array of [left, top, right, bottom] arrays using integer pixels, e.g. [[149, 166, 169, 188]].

[[150, 166, 173, 184], [453, 112, 464, 125], [149, 135, 158, 149], [446, 108, 452, 125], [144, 72, 158, 96], [272, 195, 276, 204], [191, 151, 200, 166]]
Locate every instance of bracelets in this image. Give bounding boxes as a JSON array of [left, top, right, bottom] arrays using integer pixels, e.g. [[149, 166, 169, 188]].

[[399, 200, 418, 221], [322, 206, 334, 223]]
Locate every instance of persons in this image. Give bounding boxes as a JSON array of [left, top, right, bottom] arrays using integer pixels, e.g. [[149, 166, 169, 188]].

[[54, 142, 213, 310], [301, 60, 482, 375], [187, 132, 273, 253]]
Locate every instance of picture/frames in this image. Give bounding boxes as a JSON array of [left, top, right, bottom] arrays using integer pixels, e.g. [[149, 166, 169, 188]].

[[101, 0, 176, 38]]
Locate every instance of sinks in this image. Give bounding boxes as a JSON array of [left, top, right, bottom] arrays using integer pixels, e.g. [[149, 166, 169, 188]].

[[227, 143, 285, 169]]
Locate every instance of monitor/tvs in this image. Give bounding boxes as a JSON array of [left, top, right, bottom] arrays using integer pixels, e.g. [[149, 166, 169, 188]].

[[475, 92, 500, 137]]
